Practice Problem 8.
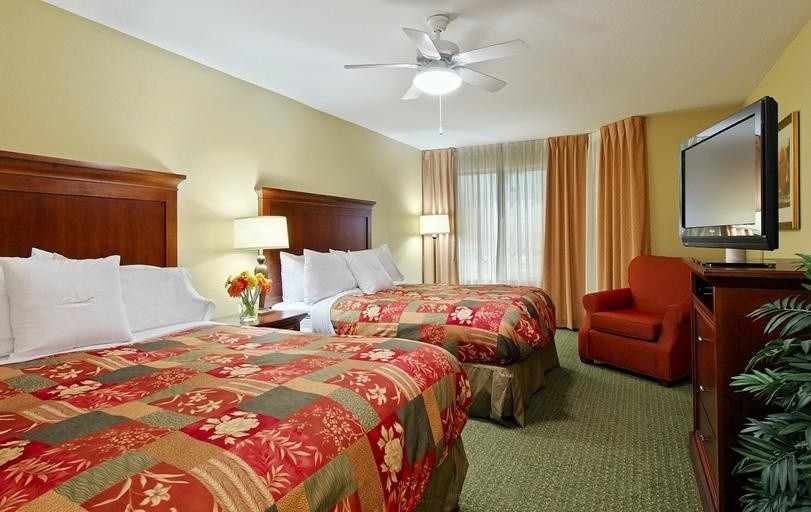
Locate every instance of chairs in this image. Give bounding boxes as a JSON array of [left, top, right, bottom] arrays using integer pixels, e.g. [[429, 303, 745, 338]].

[[576, 255, 696, 385]]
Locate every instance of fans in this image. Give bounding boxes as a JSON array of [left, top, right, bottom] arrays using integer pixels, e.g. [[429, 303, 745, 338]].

[[344, 14, 528, 101]]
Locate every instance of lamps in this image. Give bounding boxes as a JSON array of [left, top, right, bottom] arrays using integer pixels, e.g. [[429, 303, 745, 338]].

[[419, 213, 453, 282], [414, 69, 464, 137], [231, 216, 290, 314]]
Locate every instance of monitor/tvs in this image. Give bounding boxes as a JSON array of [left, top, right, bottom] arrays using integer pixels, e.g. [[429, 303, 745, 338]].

[[678, 95, 779, 268]]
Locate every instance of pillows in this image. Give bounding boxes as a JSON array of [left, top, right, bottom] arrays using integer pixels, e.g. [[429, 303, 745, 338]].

[[329, 245, 403, 289], [339, 251, 393, 293], [300, 245, 364, 303], [2, 240, 216, 371], [277, 245, 306, 304]]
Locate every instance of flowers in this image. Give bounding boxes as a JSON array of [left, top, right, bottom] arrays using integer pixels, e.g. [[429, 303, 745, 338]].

[[224, 272, 273, 318]]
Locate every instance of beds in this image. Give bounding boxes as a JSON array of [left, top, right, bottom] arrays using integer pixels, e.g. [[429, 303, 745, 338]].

[[250, 187, 565, 426], [2, 148, 475, 510]]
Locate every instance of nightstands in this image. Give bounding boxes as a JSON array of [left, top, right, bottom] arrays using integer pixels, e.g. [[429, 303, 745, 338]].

[[212, 309, 308, 332]]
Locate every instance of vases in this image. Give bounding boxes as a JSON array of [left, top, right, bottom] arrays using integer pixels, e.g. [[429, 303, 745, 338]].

[[238, 301, 262, 324]]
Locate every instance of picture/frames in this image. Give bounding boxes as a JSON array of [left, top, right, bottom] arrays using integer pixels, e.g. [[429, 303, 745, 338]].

[[777, 110, 803, 232]]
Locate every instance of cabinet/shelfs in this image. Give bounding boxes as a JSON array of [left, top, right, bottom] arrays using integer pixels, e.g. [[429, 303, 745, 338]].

[[690, 258, 811, 510]]
